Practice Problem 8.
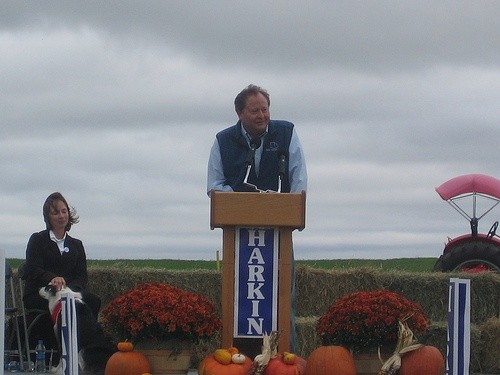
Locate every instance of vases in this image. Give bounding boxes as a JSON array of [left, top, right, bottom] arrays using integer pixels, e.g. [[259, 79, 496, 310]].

[[131, 338, 192, 375], [354, 346, 391, 375]]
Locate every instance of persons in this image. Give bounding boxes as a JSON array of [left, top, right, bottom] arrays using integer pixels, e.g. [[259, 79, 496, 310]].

[[207, 83, 308, 354], [22, 192, 110, 375]]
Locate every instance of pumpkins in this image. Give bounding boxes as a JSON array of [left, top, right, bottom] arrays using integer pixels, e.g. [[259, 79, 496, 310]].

[[104, 338, 151, 375], [197, 345, 306, 375], [304, 345, 356, 375], [397, 345, 446, 375]]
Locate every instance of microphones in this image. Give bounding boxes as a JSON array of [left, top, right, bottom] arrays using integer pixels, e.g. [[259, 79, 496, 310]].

[[236, 136, 262, 186], [277, 146, 288, 179]]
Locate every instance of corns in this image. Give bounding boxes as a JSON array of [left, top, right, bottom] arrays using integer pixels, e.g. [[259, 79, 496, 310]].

[[378, 367, 399, 375], [247, 362, 258, 375], [254, 367, 264, 375]]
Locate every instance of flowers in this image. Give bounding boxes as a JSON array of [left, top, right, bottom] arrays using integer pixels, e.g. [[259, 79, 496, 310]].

[[98, 284, 222, 355], [315, 290, 432, 352]]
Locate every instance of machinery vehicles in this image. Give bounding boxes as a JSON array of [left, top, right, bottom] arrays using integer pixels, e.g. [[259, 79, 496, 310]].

[[431, 173, 500, 275]]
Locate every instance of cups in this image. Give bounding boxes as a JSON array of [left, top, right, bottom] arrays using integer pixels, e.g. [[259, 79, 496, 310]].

[[187, 369, 198, 375]]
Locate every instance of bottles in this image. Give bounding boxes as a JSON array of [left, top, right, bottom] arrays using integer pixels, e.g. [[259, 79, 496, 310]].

[[5, 361, 34, 372], [35, 340, 46, 373]]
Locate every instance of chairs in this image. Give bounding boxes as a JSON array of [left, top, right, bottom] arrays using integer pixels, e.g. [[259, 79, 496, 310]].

[[5, 262, 57, 370]]
